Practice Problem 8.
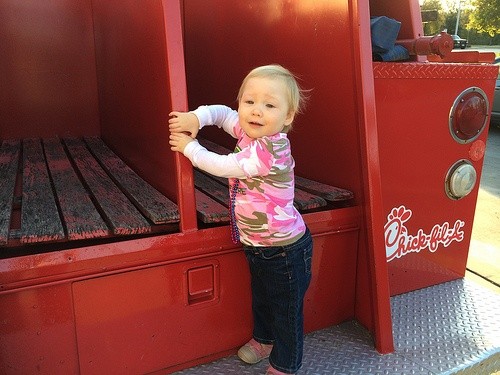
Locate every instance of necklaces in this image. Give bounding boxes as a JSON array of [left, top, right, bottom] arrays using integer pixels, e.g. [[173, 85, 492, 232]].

[[228, 178, 240, 244]]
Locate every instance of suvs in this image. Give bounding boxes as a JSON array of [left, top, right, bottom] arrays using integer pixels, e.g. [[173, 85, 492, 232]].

[[450, 35, 468, 49]]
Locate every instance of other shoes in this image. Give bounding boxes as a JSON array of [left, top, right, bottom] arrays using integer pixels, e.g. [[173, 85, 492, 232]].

[[265, 365, 295, 375], [237, 338, 274, 364]]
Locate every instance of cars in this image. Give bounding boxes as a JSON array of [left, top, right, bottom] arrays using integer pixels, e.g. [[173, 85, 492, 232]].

[[490, 56, 500, 123]]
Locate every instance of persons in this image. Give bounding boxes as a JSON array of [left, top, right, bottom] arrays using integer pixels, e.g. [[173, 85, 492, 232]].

[[168, 63, 313, 375]]
